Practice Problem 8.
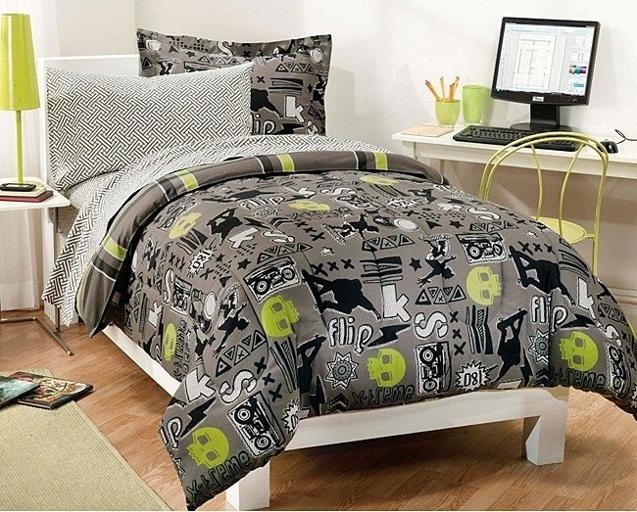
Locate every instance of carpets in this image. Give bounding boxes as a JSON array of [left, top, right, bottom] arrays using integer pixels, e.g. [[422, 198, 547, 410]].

[[0, 367, 174, 510]]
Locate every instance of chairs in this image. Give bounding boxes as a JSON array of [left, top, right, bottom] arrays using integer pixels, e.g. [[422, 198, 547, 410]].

[[479, 131, 609, 276]]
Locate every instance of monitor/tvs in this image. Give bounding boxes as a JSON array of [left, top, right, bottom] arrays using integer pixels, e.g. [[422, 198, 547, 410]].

[[491, 16, 601, 131]]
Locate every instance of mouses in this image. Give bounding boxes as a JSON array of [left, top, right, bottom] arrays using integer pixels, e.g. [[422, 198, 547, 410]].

[[597, 139, 618, 153]]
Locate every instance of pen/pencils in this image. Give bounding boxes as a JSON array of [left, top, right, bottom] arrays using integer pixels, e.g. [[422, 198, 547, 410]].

[[424, 75, 460, 101]]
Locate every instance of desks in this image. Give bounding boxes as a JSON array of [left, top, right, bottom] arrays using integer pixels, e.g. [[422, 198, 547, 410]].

[[392, 125, 637, 183]]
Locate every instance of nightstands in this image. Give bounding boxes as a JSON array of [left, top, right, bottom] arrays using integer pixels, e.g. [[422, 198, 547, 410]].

[[1, 177, 71, 356]]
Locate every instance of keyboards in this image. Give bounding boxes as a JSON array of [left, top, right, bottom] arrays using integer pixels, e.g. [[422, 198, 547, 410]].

[[452, 125, 586, 152]]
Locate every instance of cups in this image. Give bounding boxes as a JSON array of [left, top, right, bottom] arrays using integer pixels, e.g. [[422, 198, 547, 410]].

[[434, 97, 461, 130], [462, 84, 490, 125]]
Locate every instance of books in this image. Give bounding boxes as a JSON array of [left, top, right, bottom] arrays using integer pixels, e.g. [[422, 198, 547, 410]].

[[0, 185, 46, 197], [6, 371, 92, 411], [401, 123, 453, 137], [0, 190, 52, 202], [0, 375, 39, 410]]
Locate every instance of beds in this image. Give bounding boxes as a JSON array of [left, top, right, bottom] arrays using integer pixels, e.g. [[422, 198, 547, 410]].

[[34, 54, 569, 509]]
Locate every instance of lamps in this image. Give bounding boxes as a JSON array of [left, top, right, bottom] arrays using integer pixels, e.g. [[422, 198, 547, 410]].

[[1, 14, 53, 201]]
